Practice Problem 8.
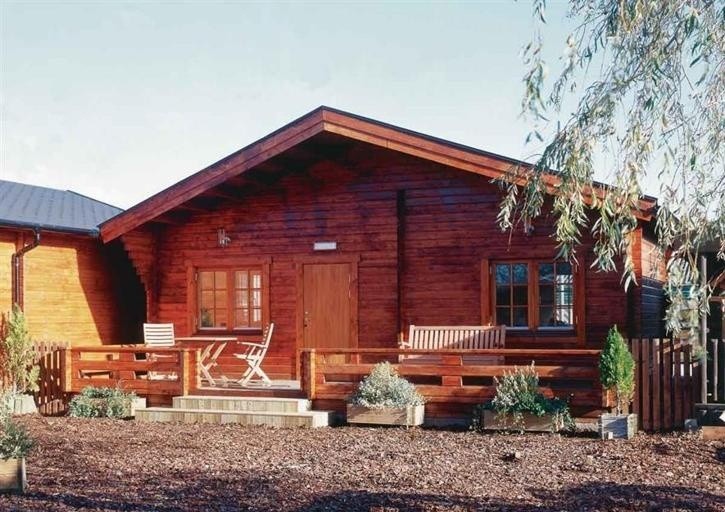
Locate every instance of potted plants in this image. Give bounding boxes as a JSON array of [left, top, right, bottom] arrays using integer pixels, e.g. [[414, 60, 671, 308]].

[[482, 359, 574, 435], [346, 360, 426, 430], [71, 377, 147, 418], [0, 302, 40, 492], [598, 323, 636, 439]]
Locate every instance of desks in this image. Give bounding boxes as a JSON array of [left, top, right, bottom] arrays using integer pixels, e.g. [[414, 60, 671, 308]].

[[174, 336, 237, 387]]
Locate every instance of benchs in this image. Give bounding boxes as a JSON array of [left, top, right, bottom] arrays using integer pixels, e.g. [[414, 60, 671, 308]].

[[397, 323, 507, 384]]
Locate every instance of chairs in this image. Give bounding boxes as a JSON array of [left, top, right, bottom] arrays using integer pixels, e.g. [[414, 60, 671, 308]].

[[142, 322, 178, 381], [232, 322, 275, 387]]
